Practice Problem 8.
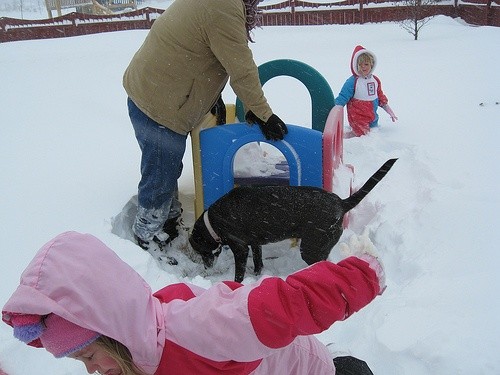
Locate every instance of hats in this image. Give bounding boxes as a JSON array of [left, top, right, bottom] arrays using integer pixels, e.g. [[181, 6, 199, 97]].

[[11, 312, 101, 359]]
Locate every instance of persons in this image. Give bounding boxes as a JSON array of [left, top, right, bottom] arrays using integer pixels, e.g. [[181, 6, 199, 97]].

[[122, 0, 289, 266], [335, 45, 399, 137], [0, 230, 389, 374]]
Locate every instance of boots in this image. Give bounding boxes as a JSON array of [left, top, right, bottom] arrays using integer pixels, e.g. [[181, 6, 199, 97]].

[[134, 209, 190, 267]]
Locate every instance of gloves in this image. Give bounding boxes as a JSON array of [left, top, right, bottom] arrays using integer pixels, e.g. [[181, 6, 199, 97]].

[[341, 234, 384, 272], [382, 104, 398, 122], [245, 111, 288, 142], [211, 96, 226, 125]]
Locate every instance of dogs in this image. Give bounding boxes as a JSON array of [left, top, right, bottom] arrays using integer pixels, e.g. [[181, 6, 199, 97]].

[[189, 158, 397, 283]]
